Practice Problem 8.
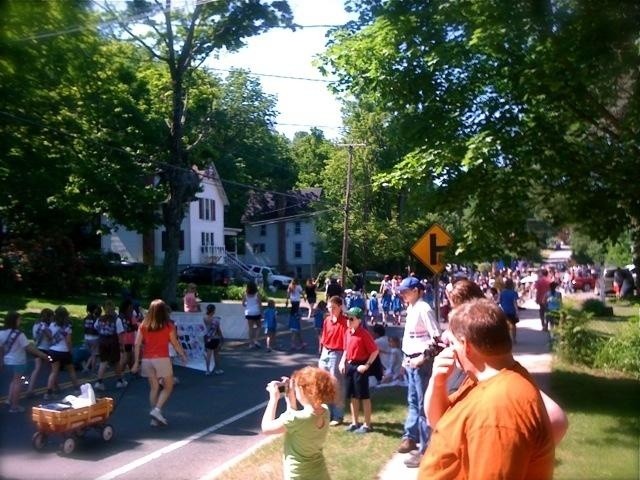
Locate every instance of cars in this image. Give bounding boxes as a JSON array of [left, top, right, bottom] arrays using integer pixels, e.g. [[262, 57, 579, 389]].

[[561, 266, 637, 299]]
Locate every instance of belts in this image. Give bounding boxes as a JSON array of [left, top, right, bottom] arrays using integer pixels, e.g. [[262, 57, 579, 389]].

[[406, 353, 422, 358], [347, 359, 366, 365], [326, 348, 335, 355]]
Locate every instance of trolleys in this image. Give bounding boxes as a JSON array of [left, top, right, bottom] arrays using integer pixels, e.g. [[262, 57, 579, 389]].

[[31, 378, 131, 455]]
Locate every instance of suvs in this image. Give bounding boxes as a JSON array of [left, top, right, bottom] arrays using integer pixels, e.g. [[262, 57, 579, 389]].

[[245, 264, 293, 289]]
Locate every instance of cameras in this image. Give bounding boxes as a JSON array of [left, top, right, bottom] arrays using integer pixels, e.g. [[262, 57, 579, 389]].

[[278, 383, 285, 399], [424, 335, 447, 360]]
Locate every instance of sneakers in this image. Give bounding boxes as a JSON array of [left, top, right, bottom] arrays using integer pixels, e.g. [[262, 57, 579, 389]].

[[5, 380, 128, 413], [205, 369, 224, 377], [150, 418, 169, 427], [345, 424, 360, 431], [354, 425, 373, 434], [329, 419, 342, 426], [249, 341, 308, 353], [150, 407, 168, 425], [403, 452, 424, 468]]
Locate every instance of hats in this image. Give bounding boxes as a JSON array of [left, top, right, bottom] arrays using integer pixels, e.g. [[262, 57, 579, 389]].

[[344, 307, 364, 320], [395, 277, 421, 291]]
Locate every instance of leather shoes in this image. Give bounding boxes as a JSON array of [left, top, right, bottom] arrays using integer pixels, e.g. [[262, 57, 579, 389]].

[[397, 439, 417, 453]]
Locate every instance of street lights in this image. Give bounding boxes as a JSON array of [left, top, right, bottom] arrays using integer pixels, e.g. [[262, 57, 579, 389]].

[[336, 143, 365, 287]]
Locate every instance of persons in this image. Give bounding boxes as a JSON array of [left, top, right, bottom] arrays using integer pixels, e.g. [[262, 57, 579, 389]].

[[0, 312, 49, 413], [242, 255, 633, 392], [338, 307, 381, 434], [43, 307, 82, 400], [448, 331, 569, 446], [205, 304, 225, 376], [260, 366, 336, 480], [25, 309, 61, 399], [416, 298, 555, 480], [130, 299, 187, 426], [184, 282, 199, 315], [393, 276, 442, 468], [82, 301, 145, 390], [318, 296, 348, 426]]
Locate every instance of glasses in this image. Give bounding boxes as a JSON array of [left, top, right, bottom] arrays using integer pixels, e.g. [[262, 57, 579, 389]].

[[344, 316, 354, 321]]
